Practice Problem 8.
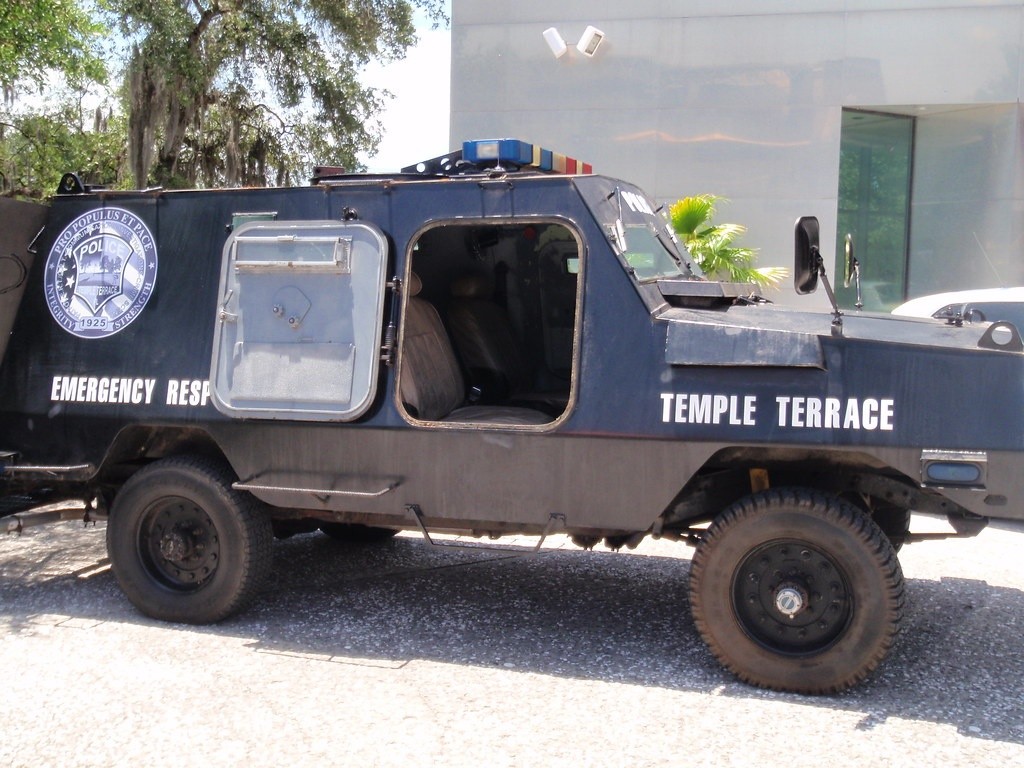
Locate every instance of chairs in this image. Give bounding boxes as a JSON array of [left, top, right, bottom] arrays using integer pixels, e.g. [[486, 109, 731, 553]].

[[446, 270, 569, 418], [399, 273, 552, 431]]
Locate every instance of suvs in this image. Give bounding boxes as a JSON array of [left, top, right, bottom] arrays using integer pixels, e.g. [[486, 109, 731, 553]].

[[1, 141, 1024, 704]]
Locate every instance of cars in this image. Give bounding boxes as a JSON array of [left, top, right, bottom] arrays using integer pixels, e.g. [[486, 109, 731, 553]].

[[886, 286, 1024, 343]]
